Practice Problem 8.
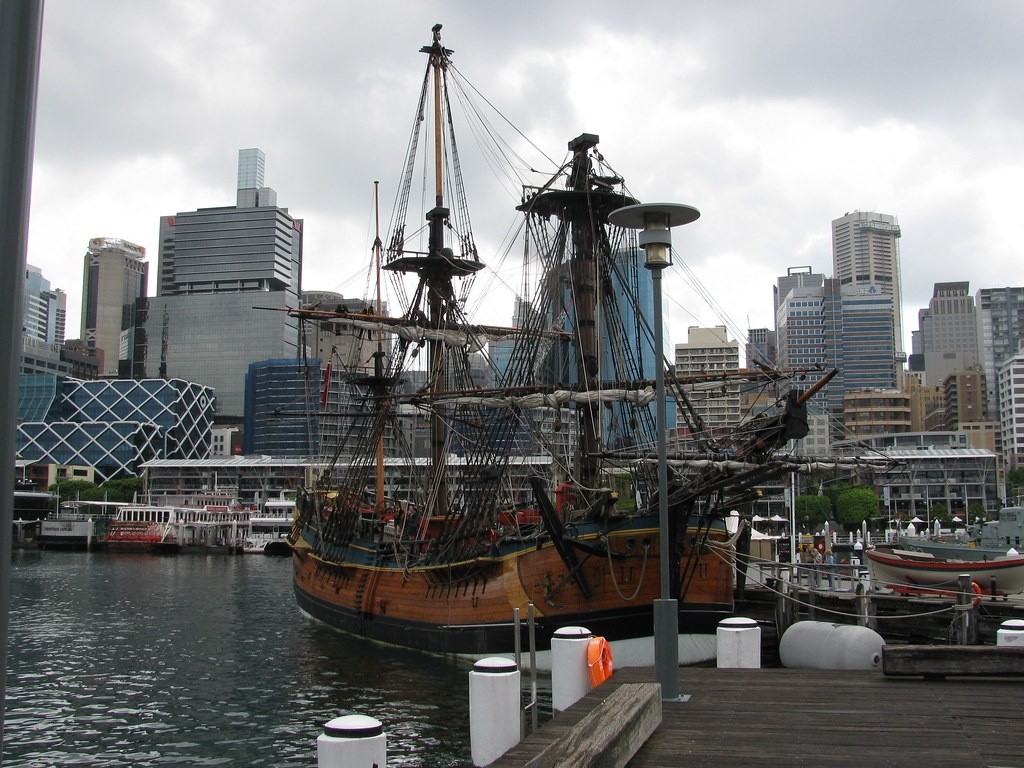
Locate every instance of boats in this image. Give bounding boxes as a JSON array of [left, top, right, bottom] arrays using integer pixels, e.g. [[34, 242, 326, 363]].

[[40, 471, 296, 554], [866, 507, 1024, 596]]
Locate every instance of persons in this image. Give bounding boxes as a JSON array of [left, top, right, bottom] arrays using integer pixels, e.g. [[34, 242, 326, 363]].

[[812, 549, 823, 587], [407, 505, 421, 543], [825, 549, 834, 589]]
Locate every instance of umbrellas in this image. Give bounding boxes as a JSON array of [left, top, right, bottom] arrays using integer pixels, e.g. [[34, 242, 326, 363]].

[[888, 516, 963, 532], [752, 515, 789, 531]]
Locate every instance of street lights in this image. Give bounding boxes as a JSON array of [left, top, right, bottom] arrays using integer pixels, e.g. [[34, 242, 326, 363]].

[[608, 203, 700, 700]]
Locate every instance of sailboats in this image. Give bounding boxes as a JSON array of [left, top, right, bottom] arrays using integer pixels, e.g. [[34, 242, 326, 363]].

[[251, 24, 907, 675]]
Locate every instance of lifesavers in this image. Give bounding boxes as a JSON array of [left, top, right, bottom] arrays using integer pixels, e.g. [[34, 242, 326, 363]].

[[971, 581, 981, 605], [588, 637, 613, 687]]
